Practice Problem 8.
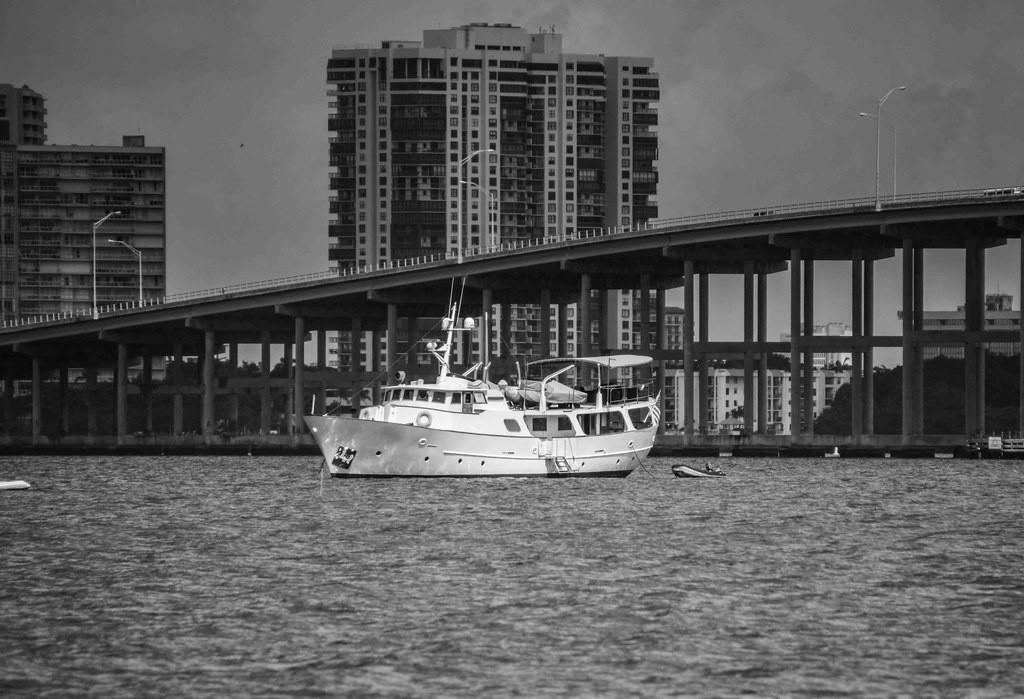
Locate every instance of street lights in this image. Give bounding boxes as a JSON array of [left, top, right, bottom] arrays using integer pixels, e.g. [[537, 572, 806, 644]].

[[92, 211, 122, 319], [108, 238, 142, 307], [860, 86, 906, 211], [456, 148, 494, 263]]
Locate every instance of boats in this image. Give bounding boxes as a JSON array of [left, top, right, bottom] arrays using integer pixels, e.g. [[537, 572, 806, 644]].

[[303, 276, 661, 479], [671, 464, 727, 478]]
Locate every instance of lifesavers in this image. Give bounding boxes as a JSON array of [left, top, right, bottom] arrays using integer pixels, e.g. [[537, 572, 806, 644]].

[[416, 411, 433, 428]]
